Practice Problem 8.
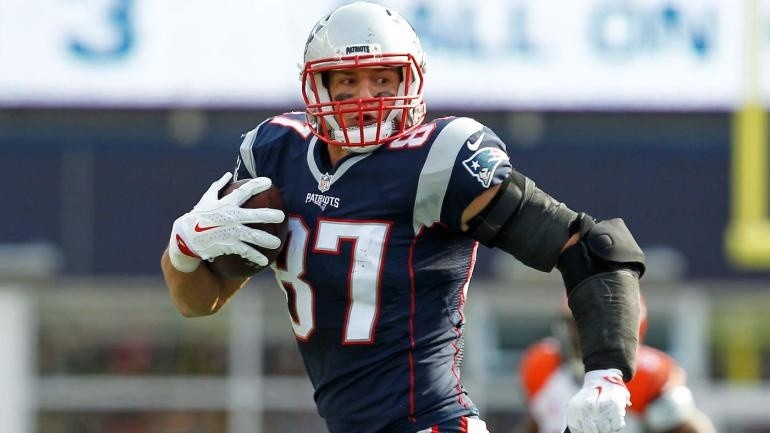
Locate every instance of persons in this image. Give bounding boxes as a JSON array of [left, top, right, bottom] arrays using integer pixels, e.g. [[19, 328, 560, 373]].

[[158, 0, 648, 432], [518, 269, 719, 431]]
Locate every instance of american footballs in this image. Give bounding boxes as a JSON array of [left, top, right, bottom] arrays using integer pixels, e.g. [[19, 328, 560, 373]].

[[199, 178, 288, 278]]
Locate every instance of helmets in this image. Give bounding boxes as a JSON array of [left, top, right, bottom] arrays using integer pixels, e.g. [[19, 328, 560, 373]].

[[301, 2, 425, 153]]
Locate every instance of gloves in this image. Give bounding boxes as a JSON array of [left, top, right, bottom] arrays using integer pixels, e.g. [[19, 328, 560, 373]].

[[567, 369, 632, 433], [170, 172, 285, 274]]
